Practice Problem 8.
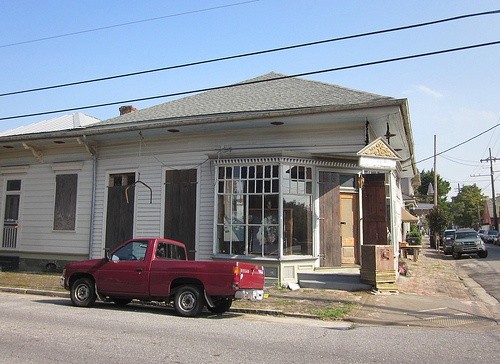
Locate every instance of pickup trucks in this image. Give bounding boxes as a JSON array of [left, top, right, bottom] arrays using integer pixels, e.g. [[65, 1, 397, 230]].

[[60, 237, 265, 318]]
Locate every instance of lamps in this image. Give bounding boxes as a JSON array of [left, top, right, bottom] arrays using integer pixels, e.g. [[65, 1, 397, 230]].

[[357, 174, 365, 188]]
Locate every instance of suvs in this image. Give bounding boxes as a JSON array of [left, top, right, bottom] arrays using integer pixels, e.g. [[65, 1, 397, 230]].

[[441, 228, 500, 259]]
